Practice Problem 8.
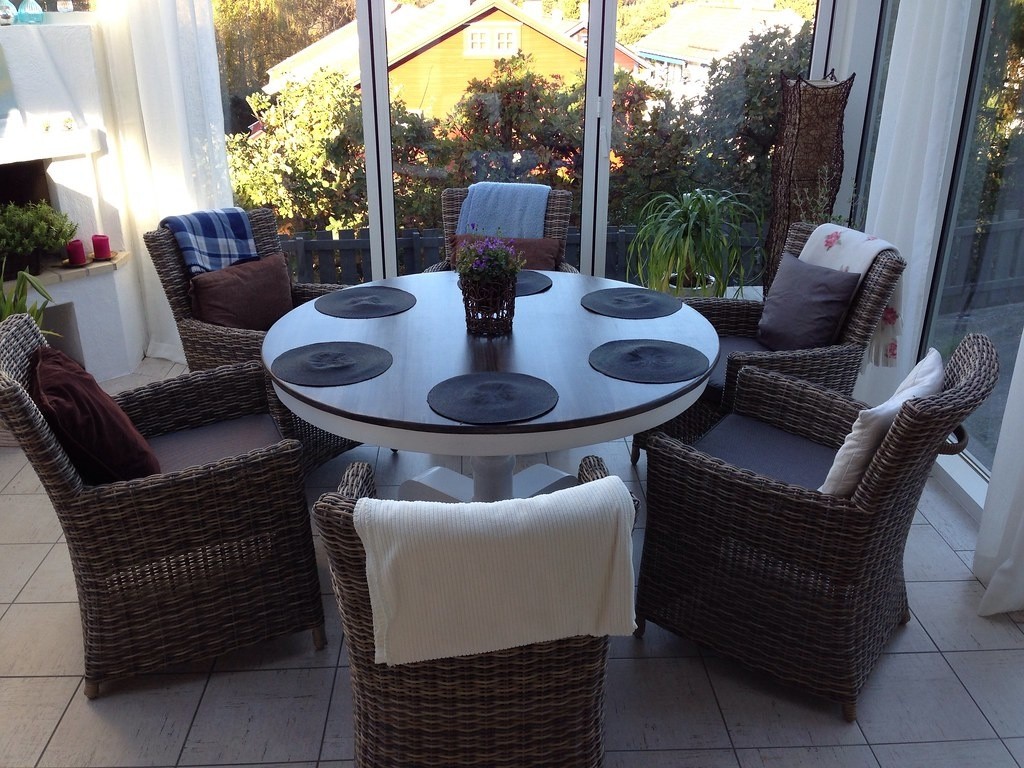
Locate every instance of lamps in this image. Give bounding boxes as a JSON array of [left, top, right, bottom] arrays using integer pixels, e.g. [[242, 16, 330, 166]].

[[762, 67, 856, 301]]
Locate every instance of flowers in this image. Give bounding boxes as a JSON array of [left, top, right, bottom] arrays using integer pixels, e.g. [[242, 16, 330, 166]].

[[453, 239, 528, 277]]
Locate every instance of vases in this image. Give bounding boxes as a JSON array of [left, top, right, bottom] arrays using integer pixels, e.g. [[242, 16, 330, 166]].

[[458, 273, 518, 339]]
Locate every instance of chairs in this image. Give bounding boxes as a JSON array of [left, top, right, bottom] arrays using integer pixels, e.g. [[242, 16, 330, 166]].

[[0, 313, 327, 698], [305, 457, 640, 768], [143, 207, 345, 368], [631, 335, 999, 727], [421, 181, 580, 277], [680, 221, 904, 404]]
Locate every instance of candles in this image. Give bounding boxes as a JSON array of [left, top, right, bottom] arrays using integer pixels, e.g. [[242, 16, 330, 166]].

[[92, 234, 112, 259], [65, 239, 86, 265]]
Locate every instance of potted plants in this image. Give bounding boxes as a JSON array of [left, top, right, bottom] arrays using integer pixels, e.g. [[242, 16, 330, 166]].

[[0, 196, 79, 281], [624, 186, 771, 301]]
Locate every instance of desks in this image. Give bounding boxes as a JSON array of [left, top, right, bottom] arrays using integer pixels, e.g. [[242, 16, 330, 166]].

[[258, 267, 719, 506]]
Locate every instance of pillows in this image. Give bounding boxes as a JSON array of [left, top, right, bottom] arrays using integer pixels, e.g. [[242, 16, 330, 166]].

[[449, 231, 561, 273], [755, 250, 860, 351], [822, 348, 942, 494], [192, 248, 292, 333], [35, 343, 160, 486]]
